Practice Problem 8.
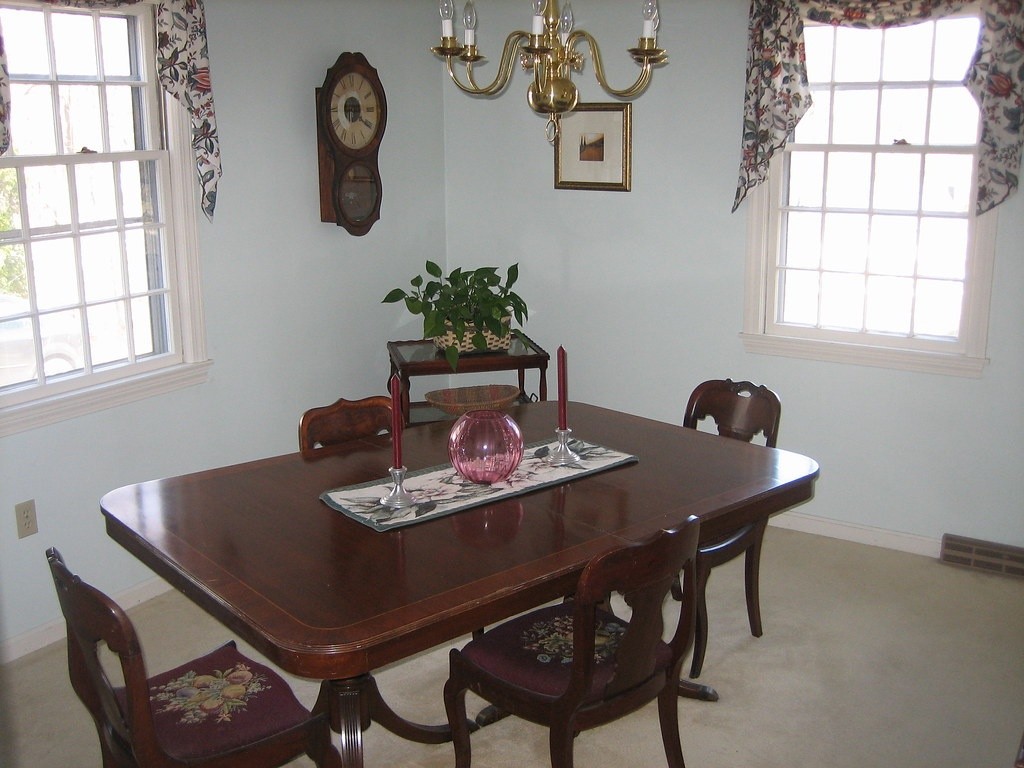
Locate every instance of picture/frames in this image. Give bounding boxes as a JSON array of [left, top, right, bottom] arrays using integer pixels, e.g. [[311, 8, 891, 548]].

[[553, 103, 631, 192]]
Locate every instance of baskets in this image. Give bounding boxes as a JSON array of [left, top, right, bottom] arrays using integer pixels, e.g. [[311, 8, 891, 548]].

[[425, 385, 521, 417], [433, 304, 512, 353]]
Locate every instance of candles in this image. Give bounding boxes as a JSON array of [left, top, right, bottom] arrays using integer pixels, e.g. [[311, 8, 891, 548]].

[[391, 373, 402, 469], [557, 343, 568, 430]]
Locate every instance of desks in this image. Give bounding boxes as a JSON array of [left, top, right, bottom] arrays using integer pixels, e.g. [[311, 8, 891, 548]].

[[102, 400, 818, 768], [387, 328, 550, 427]]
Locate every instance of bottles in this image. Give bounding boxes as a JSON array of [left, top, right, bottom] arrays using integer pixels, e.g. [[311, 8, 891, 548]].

[[447, 409, 525, 484]]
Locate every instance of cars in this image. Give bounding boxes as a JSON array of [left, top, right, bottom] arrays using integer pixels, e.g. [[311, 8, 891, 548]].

[[0, 292, 86, 386]]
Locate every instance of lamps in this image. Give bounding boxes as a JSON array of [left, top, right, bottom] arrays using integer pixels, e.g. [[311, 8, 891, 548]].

[[429, 0, 668, 144]]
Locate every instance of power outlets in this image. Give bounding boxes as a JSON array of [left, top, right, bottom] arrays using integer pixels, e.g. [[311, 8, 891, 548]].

[[15, 498, 38, 540]]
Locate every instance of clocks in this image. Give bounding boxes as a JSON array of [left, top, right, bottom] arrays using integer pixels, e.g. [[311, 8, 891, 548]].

[[315, 49, 387, 236]]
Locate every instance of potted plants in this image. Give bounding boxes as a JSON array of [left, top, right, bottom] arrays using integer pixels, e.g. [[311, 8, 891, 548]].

[[381, 260, 528, 372]]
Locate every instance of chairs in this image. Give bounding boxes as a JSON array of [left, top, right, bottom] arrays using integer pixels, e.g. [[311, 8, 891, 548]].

[[45, 545, 341, 768], [444, 514, 701, 768], [298, 395, 405, 452], [671, 378, 782, 638]]
